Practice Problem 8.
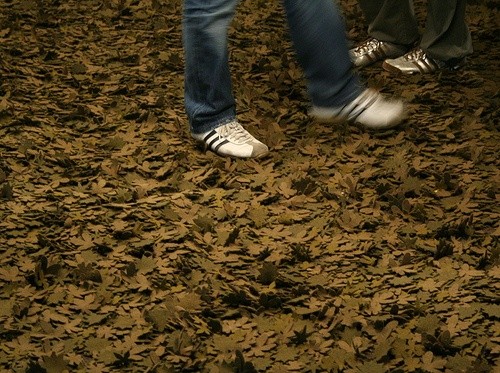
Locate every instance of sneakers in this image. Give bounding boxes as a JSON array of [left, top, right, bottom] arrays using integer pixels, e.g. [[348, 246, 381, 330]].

[[347, 38, 421, 68], [190, 119, 269, 159], [310, 88, 404, 128], [382, 45, 467, 75]]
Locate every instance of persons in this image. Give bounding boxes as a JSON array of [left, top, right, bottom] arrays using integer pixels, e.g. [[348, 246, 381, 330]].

[[181, 0, 403, 160], [346, 0, 476, 76]]
